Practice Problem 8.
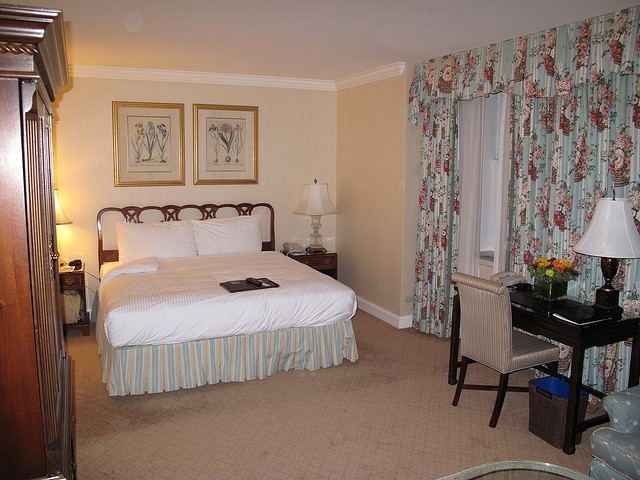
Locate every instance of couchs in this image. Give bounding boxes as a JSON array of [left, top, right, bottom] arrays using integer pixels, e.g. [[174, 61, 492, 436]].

[[589, 386, 639, 480]]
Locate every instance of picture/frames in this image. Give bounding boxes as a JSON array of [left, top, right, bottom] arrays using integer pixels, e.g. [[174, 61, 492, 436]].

[[193, 103, 259, 185], [112, 100, 186, 186]]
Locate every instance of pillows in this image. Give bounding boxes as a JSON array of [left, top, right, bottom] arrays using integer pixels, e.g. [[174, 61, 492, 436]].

[[115, 221, 197, 264], [196, 213, 262, 256]]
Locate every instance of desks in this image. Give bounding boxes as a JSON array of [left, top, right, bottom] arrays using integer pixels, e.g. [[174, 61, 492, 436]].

[[448, 286, 640, 455]]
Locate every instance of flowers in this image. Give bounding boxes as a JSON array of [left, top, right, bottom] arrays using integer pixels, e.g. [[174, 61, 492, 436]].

[[527, 256, 580, 296]]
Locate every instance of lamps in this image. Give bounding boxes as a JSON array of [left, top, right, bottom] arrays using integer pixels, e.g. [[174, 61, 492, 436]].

[[572, 198, 640, 321], [292, 179, 339, 254], [54, 188, 73, 269]]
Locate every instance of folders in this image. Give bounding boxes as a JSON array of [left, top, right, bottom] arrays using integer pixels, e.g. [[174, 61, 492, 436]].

[[554, 305, 612, 326]]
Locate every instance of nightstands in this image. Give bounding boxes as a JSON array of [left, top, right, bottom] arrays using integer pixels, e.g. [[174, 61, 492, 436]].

[[282, 250, 337, 283], [59, 262, 90, 337]]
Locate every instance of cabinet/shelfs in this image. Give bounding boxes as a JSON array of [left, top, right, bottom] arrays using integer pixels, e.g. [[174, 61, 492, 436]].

[[0, 4, 76, 480]]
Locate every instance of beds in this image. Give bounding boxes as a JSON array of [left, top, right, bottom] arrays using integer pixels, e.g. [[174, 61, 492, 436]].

[[97, 202, 359, 396]]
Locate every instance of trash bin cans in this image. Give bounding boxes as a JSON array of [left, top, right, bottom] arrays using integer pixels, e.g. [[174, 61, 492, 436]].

[[529, 377, 589, 449]]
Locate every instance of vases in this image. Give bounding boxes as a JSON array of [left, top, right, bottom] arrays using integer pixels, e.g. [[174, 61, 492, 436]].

[[532, 278, 568, 303]]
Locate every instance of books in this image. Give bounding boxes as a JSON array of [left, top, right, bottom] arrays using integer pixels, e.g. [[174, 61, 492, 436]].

[[551, 303, 615, 328]]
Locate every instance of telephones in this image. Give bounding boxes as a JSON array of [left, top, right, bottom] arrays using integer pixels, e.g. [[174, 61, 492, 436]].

[[283, 243, 302, 256], [490, 271, 526, 287]]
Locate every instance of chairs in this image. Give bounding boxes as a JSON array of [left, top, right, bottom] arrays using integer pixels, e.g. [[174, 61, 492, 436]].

[[451, 272, 560, 428]]
